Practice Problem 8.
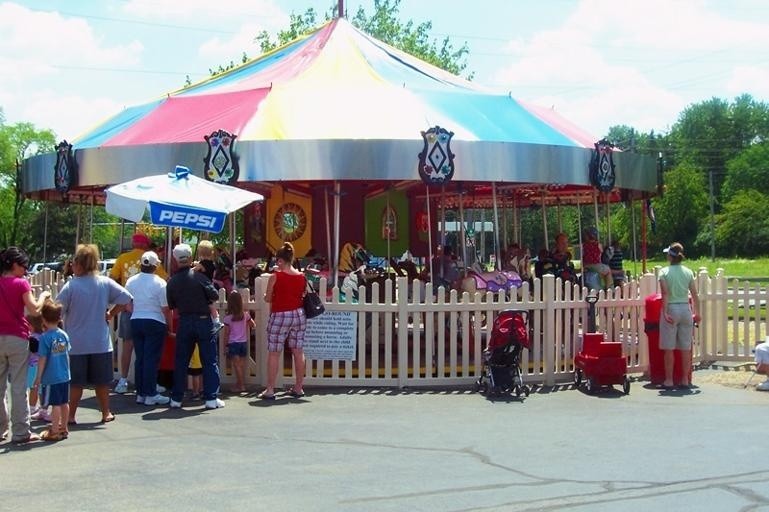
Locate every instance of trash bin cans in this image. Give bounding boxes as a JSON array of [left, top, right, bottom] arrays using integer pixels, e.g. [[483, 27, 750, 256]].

[[644, 294, 693, 386]]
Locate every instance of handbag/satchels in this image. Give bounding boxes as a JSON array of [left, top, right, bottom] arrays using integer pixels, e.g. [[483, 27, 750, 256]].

[[304, 287, 325, 318]]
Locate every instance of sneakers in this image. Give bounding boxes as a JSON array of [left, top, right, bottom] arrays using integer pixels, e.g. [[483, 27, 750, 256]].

[[11, 405, 76, 442], [190, 392, 225, 409], [115, 381, 128, 393], [210, 323, 224, 335], [136, 384, 183, 408]]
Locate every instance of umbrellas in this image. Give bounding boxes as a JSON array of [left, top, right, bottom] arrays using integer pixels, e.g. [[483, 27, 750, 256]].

[[104, 163, 265, 244]]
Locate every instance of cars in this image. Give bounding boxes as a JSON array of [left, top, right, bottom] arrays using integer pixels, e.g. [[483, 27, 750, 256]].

[[27, 260, 60, 275], [98, 258, 117, 272]]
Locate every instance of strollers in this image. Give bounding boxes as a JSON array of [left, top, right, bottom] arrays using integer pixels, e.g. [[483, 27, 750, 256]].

[[474, 308, 534, 399]]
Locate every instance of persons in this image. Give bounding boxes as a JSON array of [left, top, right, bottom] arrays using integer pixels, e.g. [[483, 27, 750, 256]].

[[755, 333, 768, 390], [426, 227, 623, 289], [223, 290, 256, 397], [258, 243, 308, 399], [657, 241, 702, 389], [0, 239, 225, 443]]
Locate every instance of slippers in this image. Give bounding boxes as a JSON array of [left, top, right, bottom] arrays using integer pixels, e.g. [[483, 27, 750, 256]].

[[656, 383, 674, 390], [257, 391, 276, 400], [102, 413, 115, 422], [288, 387, 305, 397]]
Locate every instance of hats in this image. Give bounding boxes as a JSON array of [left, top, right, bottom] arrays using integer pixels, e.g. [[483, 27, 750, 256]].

[[173, 244, 192, 261], [585, 226, 598, 236], [662, 242, 685, 256], [141, 251, 161, 266], [133, 235, 149, 243]]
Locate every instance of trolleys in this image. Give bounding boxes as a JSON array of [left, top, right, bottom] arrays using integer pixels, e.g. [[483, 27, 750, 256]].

[[573, 294, 631, 395]]
